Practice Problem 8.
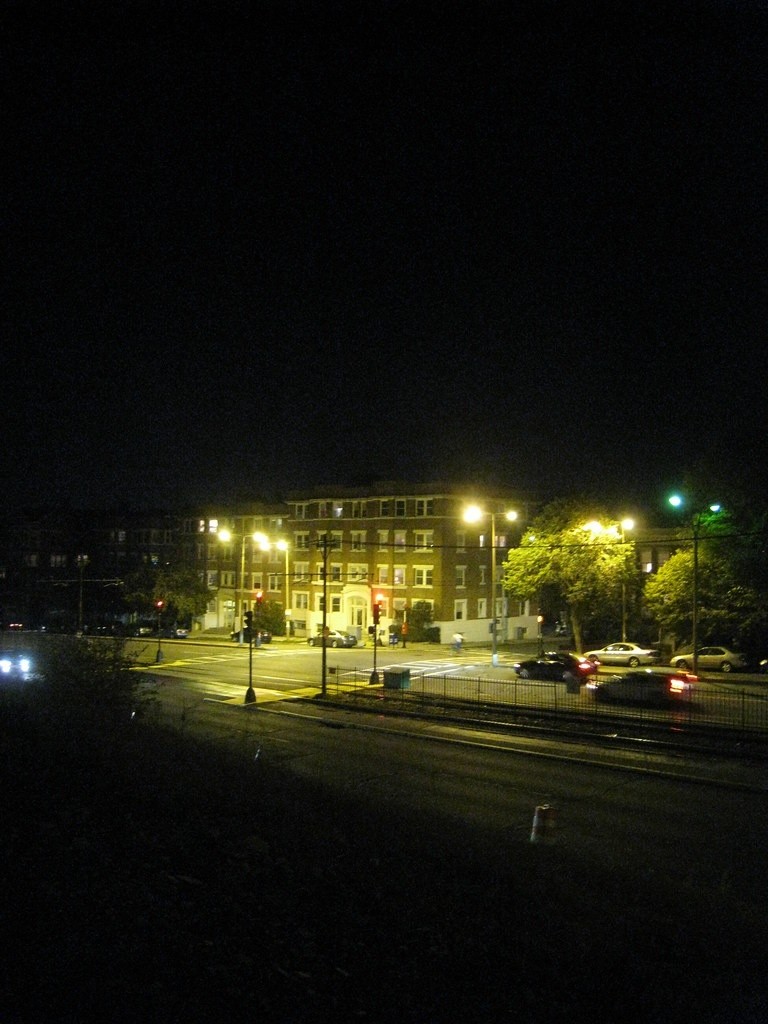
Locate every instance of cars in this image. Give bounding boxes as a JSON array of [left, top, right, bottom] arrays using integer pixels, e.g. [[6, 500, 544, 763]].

[[0, 648, 31, 675], [230, 627, 272, 644], [670, 646, 749, 673], [586, 671, 696, 709], [584, 642, 661, 668], [514, 651, 597, 683], [307, 630, 358, 648]]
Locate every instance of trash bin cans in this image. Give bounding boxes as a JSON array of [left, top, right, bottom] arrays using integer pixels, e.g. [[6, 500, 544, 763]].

[[515, 626, 524, 640], [383, 667, 411, 689], [389, 634, 398, 644]]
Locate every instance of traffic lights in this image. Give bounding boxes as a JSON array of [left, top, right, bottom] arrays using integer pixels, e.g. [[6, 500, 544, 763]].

[[374, 604, 380, 624], [377, 593, 383, 605], [157, 601, 163, 607], [664, 494, 681, 526], [244, 612, 251, 626], [257, 592, 263, 606]]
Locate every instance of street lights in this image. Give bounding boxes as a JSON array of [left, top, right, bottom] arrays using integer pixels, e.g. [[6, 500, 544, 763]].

[[463, 505, 520, 668], [621, 517, 636, 640], [74, 548, 88, 635], [682, 503, 720, 674], [217, 528, 268, 642]]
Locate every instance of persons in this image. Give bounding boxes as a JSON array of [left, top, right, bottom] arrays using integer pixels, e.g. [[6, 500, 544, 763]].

[[453, 632, 464, 650]]
